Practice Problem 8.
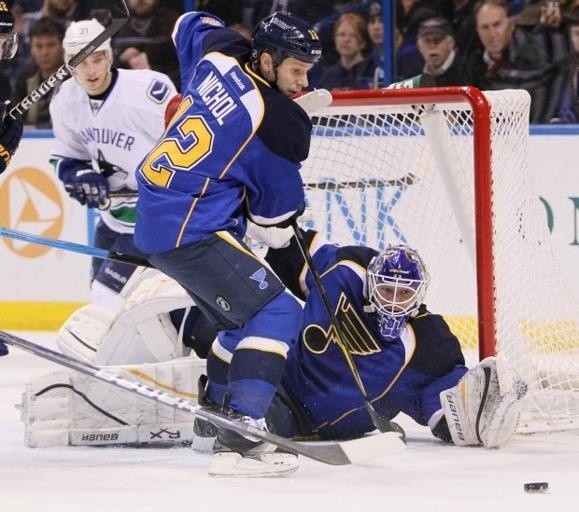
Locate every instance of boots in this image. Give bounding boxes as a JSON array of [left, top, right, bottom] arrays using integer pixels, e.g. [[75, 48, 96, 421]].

[[189, 405, 299, 459]]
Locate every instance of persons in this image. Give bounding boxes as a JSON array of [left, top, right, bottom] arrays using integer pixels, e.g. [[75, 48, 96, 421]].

[[134, 11, 323, 477], [49, 17, 181, 289], [26, 226, 530, 449], [228, 23, 252, 39], [308, 0, 579, 126], [1, 1, 38, 356], [1, 1, 186, 128]]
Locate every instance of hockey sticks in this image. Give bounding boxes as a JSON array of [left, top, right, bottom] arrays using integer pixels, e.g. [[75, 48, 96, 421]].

[[292, 221, 405, 442], [0, 331, 407, 463], [107, 145, 432, 198]]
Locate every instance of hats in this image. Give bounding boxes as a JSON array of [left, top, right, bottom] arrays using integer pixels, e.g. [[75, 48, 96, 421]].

[[417, 17, 452, 38]]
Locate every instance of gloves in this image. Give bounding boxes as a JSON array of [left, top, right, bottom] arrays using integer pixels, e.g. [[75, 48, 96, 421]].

[[64, 167, 108, 207]]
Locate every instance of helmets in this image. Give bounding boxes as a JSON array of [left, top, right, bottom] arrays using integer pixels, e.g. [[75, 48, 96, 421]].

[[0, 3, 15, 34], [60, 17, 113, 63], [252, 10, 324, 64], [369, 244, 428, 294]]
